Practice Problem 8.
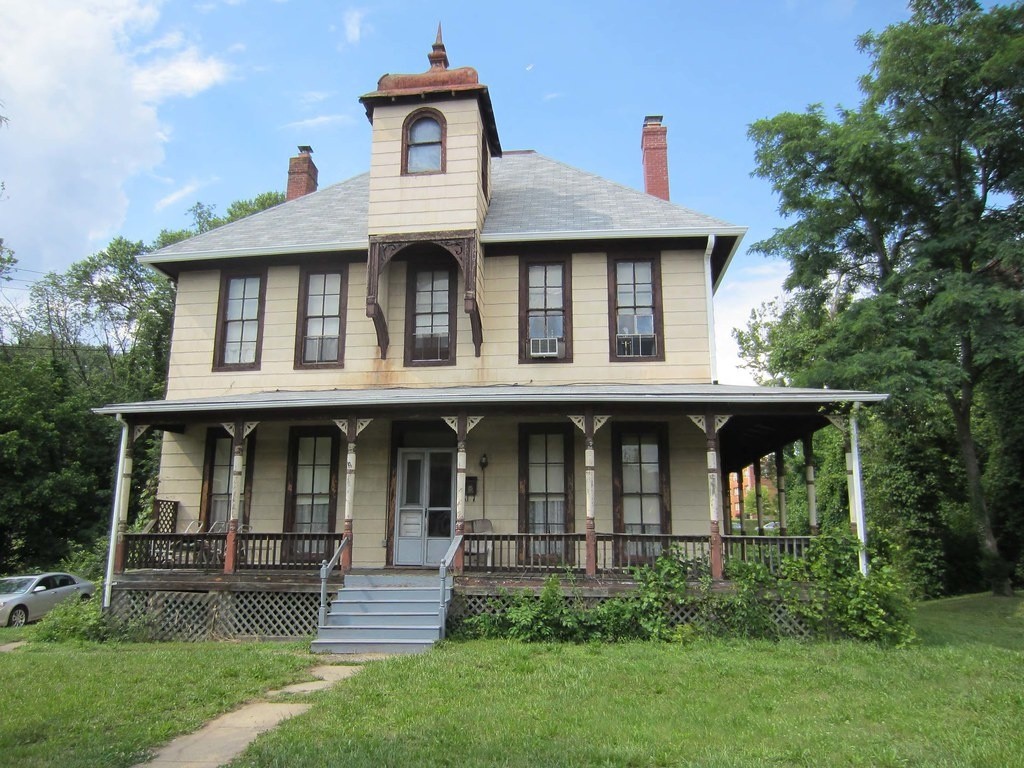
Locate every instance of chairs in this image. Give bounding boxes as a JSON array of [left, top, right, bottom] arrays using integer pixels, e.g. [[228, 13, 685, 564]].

[[464, 519, 494, 567], [166, 521, 253, 564]]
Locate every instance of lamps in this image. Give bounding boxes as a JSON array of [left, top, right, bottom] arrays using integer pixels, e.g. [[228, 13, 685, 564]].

[[480, 454, 488, 471]]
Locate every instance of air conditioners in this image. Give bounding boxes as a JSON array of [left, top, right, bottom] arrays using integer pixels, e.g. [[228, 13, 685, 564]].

[[531, 337, 559, 358]]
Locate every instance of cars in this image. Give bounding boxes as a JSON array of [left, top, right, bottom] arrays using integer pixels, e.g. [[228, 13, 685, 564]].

[[0, 573, 96, 628], [756, 521, 781, 533], [731, 522, 746, 530]]
